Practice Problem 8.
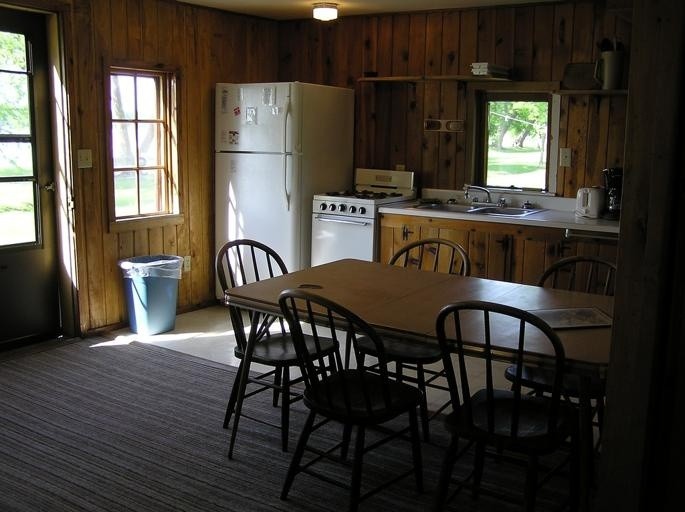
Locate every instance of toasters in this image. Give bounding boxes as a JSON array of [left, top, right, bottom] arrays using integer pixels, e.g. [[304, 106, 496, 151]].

[[576, 186, 605, 221]]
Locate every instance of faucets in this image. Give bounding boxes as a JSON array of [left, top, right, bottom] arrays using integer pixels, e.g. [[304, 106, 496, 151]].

[[464, 186, 491, 203]]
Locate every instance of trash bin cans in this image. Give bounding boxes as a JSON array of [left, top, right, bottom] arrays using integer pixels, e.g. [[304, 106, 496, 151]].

[[119, 254, 184, 335]]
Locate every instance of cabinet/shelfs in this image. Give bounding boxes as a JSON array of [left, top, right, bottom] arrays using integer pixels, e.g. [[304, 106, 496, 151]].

[[508, 224, 619, 296], [379, 214, 508, 282]]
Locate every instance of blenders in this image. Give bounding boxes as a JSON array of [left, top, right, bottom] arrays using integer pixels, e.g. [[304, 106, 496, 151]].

[[602, 167, 620, 221]]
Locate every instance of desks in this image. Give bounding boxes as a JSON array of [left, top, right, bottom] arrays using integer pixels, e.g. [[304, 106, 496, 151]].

[[225, 259, 614, 512]]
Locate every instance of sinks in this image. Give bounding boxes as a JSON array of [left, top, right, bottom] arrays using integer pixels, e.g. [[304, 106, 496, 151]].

[[405, 203, 478, 213], [467, 207, 534, 215]]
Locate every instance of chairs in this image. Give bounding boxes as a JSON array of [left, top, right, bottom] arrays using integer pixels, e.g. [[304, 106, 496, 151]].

[[279, 292, 423, 512], [436, 300, 580, 512], [496, 256, 617, 467], [355, 238, 471, 443], [216, 240, 339, 451]]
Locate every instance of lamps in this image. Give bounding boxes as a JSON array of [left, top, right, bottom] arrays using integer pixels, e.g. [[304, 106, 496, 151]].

[[313, 4, 338, 22]]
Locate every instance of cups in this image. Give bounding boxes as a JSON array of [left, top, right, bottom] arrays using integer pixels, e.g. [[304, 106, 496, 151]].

[[594, 50, 630, 90]]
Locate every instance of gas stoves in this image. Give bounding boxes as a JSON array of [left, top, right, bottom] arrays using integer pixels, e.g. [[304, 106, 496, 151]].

[[311, 168, 418, 220]]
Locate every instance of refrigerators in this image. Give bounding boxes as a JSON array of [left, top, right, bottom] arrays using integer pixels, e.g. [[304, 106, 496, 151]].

[[214, 81, 356, 309]]
